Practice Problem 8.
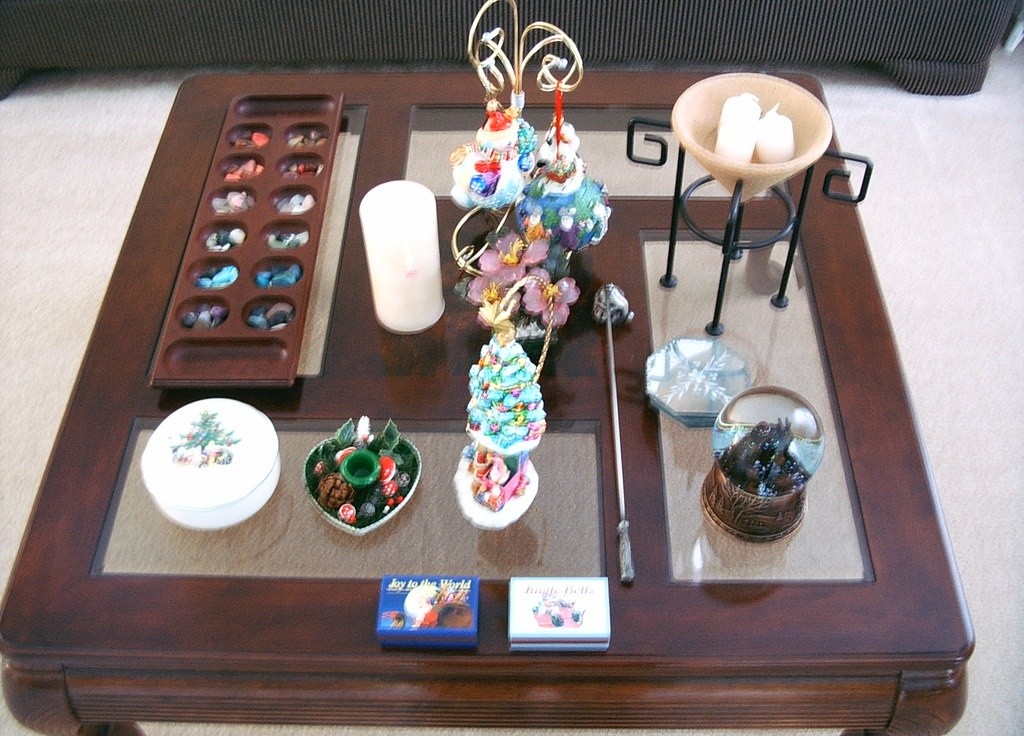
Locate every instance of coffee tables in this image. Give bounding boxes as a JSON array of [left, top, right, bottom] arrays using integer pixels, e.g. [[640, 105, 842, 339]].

[[0, 68, 975, 736]]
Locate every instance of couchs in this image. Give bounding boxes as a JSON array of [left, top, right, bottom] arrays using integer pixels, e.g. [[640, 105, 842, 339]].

[[0, 0, 1018, 100]]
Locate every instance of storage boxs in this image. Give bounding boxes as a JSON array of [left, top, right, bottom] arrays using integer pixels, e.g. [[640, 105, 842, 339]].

[[506, 575, 610, 650], [374, 573, 479, 646]]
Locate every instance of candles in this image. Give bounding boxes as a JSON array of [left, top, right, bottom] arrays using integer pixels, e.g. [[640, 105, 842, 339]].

[[714, 119, 755, 162], [757, 102, 794, 164], [720, 91, 762, 119], [359, 179, 446, 334]]
